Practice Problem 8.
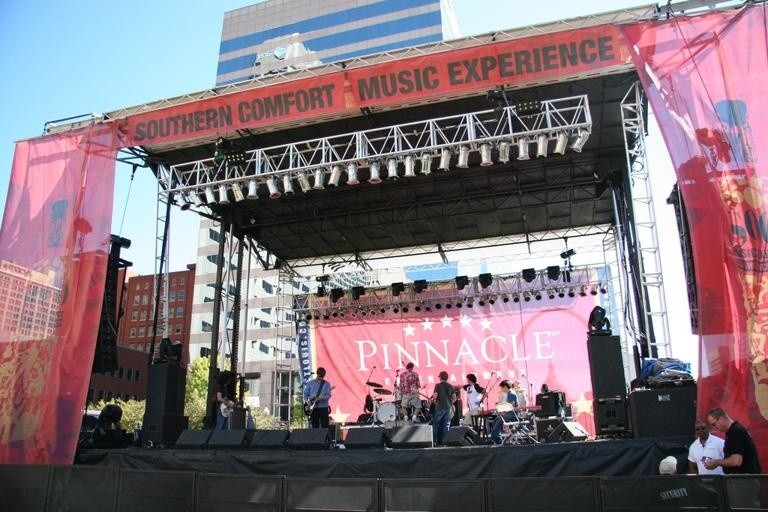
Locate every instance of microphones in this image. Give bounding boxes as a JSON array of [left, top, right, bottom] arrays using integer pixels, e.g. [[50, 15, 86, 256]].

[[373, 365, 377, 369], [310, 372, 315, 374], [421, 384, 427, 390], [395, 369, 400, 372], [401, 360, 406, 367]]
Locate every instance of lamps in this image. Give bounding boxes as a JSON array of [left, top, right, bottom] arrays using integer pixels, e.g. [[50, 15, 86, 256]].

[[292, 263, 607, 323], [368, 85, 593, 185], [585, 305, 612, 335], [157, 337, 183, 366], [168, 136, 359, 213]]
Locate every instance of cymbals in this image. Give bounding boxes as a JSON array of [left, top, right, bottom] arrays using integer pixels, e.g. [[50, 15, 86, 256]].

[[366, 382, 383, 387], [374, 389, 392, 395]]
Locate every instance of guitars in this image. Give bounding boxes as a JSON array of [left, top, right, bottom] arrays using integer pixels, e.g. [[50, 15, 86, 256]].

[[220, 401, 234, 418], [304, 385, 336, 416]]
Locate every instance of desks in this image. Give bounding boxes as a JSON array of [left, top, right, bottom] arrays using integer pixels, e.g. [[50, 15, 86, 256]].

[[536, 416, 573, 442]]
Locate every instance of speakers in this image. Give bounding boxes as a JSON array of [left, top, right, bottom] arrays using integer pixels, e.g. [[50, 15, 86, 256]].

[[176, 428, 213, 449], [143, 415, 190, 449], [547, 421, 589, 442], [288, 428, 332, 451], [444, 425, 485, 445], [628, 388, 690, 438], [586, 335, 627, 398], [254, 429, 289, 451], [208, 429, 252, 451], [591, 398, 628, 437], [674, 183, 749, 334], [343, 428, 384, 450], [389, 426, 434, 448], [56, 258, 119, 373], [142, 365, 187, 415]]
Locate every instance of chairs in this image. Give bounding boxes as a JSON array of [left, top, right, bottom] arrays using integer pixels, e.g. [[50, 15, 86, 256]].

[[495, 401, 539, 445]]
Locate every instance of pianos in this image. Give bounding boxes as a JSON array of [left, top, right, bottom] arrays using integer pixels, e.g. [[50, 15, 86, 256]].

[[481, 406, 542, 417]]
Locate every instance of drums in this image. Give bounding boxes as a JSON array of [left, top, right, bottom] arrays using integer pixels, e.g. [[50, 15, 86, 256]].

[[376, 401, 403, 424], [412, 400, 429, 417]]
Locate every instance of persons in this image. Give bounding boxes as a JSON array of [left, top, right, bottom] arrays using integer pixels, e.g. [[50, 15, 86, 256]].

[[486, 380, 530, 445], [433, 370, 457, 447], [302, 367, 332, 429], [688, 421, 725, 476], [214, 375, 235, 430], [399, 363, 421, 424], [704, 409, 761, 475], [455, 375, 487, 439]]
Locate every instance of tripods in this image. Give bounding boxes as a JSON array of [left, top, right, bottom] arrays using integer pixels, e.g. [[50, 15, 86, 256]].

[[410, 404, 428, 423]]
